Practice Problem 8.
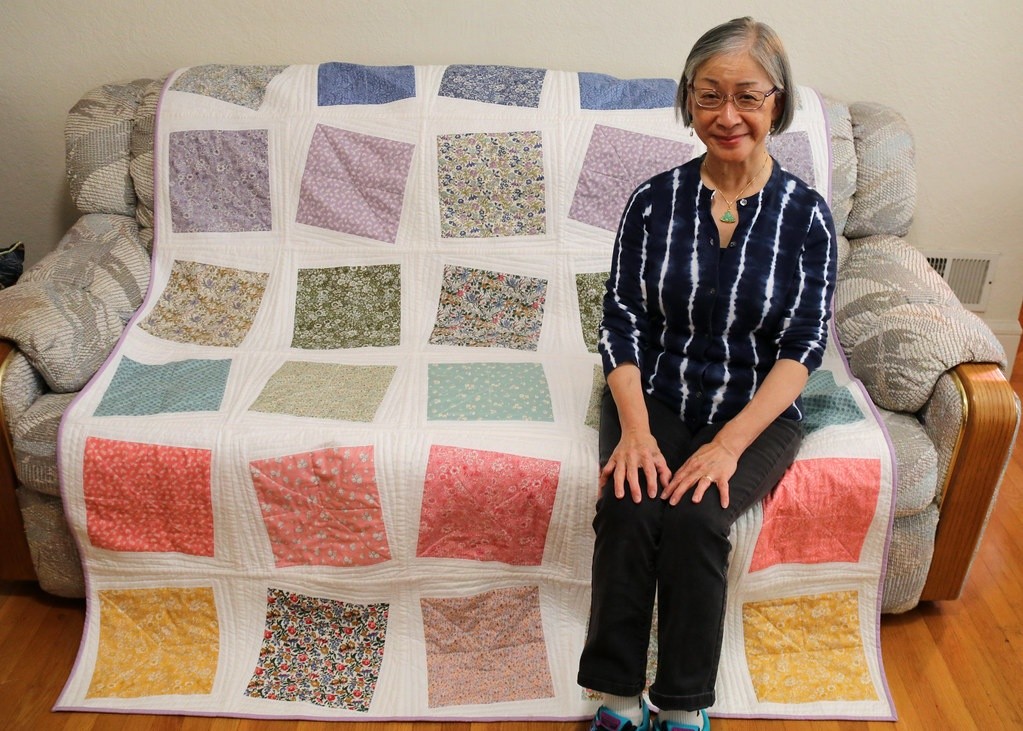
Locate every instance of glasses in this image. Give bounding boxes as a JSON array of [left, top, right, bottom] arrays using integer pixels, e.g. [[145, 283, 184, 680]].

[[691, 80, 778, 110]]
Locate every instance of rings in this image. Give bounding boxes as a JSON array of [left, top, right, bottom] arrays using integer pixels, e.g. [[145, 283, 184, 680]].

[[701, 474, 713, 482]]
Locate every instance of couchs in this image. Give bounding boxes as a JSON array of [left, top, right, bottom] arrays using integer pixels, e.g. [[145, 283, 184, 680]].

[[0, 61, 1022, 614]]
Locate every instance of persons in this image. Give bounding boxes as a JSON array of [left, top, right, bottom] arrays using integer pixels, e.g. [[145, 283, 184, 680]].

[[572, 12, 838, 731]]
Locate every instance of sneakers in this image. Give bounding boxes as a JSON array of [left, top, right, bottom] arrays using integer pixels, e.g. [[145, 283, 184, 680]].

[[589, 699, 651, 731], [652, 708, 711, 731]]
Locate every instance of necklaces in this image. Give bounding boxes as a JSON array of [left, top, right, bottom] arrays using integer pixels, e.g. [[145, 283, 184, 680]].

[[701, 151, 772, 224]]
